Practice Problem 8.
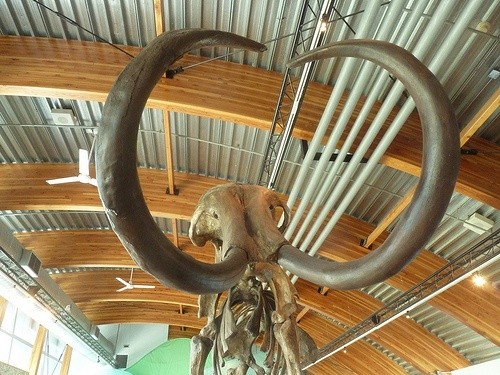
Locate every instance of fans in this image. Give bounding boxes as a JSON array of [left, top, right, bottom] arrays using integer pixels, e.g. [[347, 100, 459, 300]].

[[114, 269, 156, 292], [46, 128, 98, 186]]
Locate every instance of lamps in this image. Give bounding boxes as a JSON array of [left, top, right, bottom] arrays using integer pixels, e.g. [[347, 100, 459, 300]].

[[319, 13, 329, 32]]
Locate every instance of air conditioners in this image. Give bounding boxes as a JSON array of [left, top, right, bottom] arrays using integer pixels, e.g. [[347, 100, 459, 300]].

[[19, 248, 42, 278], [89, 324, 101, 340]]
